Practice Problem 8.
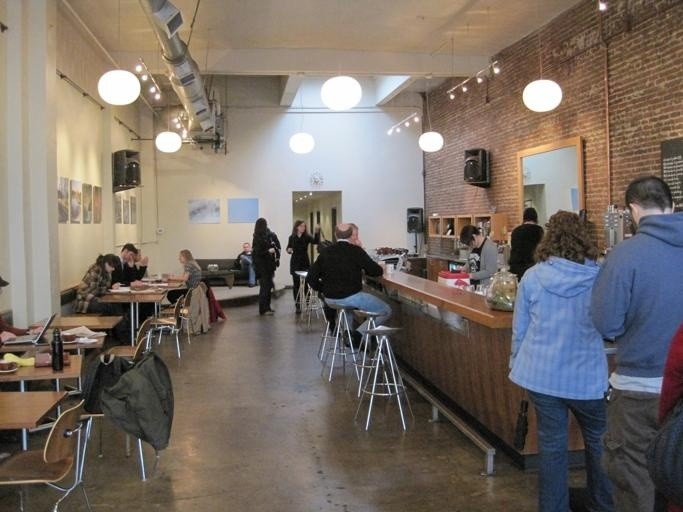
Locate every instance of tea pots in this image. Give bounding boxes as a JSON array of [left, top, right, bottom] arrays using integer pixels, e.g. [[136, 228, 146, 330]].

[[485, 264, 519, 312]]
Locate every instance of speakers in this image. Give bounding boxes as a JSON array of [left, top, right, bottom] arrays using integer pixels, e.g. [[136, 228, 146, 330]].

[[407, 207, 423, 233], [112, 149, 141, 193], [463, 148, 490, 189]]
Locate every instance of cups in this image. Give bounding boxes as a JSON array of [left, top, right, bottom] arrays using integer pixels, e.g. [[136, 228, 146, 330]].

[[463, 283, 488, 296], [376, 247, 391, 255], [391, 247, 408, 254], [432, 213, 439, 217], [61, 332, 75, 341], [0, 360, 16, 371], [385, 264, 394, 274], [377, 261, 385, 264]]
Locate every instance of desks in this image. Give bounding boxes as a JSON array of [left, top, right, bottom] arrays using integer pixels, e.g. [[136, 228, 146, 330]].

[[0, 277, 205, 512]]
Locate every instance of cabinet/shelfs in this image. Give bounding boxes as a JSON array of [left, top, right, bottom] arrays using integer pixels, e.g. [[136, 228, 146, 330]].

[[426, 212, 508, 281]]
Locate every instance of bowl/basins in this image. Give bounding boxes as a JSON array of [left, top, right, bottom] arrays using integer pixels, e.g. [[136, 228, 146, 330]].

[[486, 208, 496, 214]]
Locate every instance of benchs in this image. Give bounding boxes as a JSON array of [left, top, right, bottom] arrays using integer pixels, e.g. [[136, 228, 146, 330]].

[[194, 259, 260, 289]]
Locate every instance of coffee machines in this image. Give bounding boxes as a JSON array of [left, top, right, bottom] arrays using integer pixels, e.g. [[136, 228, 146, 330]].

[[600, 210, 635, 253]]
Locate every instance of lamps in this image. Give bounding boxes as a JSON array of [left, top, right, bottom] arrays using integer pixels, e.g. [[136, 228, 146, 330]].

[[155, 83, 182, 153], [449, 66, 501, 100], [418, 76, 444, 153], [97, 0, 141, 106], [320, 56, 362, 112], [289, 86, 315, 154], [136, 57, 161, 101], [522, 0, 563, 113], [174, 117, 188, 139], [387, 115, 420, 138]]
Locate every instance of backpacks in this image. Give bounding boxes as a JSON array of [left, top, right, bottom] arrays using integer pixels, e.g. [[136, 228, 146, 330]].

[[81, 354, 135, 414]]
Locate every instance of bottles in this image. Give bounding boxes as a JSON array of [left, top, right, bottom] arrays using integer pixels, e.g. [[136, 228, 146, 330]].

[[446, 223, 453, 235], [608, 205, 617, 212], [51, 328, 63, 370]]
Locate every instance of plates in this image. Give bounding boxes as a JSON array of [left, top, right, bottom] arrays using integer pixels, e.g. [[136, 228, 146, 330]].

[[62, 339, 78, 344], [0, 367, 17, 374]]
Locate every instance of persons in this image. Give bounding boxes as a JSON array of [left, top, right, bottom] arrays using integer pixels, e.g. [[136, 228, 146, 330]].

[[167, 249, 201, 304], [508, 206, 544, 280], [651, 321, 683, 510], [133, 249, 148, 281], [74, 253, 132, 346], [286, 219, 322, 314], [1, 276, 48, 348], [507, 211, 613, 511], [589, 176, 682, 511], [306, 223, 392, 354], [235, 242, 257, 287], [459, 223, 500, 294], [109, 243, 138, 287], [251, 218, 281, 315]]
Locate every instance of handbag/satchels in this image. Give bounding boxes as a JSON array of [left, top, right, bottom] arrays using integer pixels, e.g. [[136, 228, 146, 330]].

[[485, 272, 519, 311], [317, 240, 333, 253]]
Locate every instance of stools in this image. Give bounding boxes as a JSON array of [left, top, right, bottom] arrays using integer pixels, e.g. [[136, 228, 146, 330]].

[[291, 271, 415, 430]]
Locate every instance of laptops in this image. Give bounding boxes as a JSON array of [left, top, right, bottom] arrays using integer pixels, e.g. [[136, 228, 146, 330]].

[[4, 311, 57, 345]]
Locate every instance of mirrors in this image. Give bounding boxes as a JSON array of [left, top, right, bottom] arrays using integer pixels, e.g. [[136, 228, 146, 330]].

[[516, 136, 584, 231]]
